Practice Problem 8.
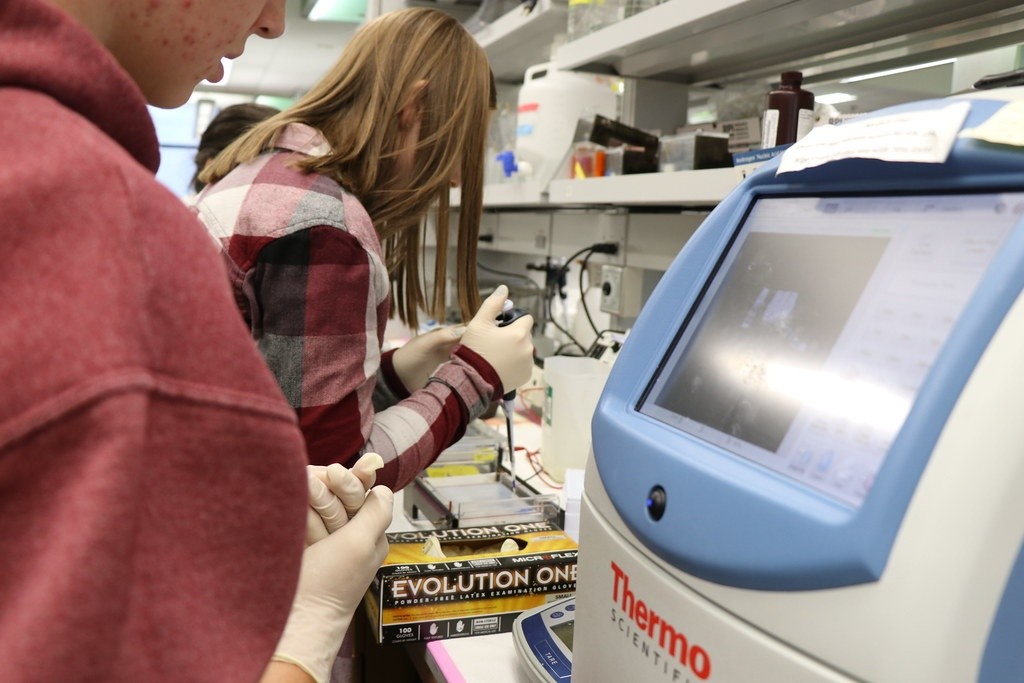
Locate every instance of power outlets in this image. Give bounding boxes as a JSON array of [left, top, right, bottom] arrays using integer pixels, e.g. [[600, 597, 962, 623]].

[[597, 213, 629, 267]]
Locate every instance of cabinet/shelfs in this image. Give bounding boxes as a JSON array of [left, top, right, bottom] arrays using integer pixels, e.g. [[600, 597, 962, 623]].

[[406, 0, 1024, 682]]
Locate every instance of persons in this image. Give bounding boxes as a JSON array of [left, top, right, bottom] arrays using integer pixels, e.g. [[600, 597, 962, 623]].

[[0, 0, 394, 683], [186, 7, 534, 683]]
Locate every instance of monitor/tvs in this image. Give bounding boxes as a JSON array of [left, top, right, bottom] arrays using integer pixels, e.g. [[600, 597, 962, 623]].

[[634, 194, 1024, 507]]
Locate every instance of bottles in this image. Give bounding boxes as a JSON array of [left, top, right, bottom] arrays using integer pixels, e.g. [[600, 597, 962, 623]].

[[758, 72, 814, 147]]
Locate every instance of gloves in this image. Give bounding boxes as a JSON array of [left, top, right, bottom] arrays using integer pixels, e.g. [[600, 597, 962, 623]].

[[392, 322, 467, 395], [269, 453, 396, 683], [459, 284, 535, 396]]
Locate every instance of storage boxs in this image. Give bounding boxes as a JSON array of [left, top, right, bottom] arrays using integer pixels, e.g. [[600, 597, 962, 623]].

[[677, 116, 763, 145]]
